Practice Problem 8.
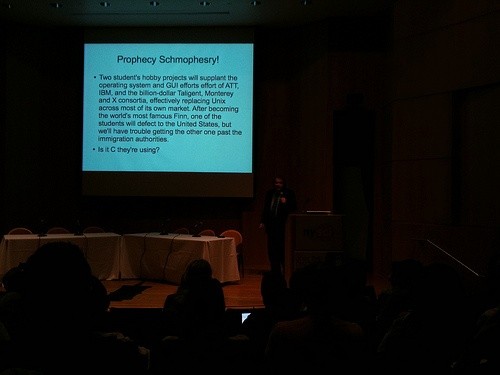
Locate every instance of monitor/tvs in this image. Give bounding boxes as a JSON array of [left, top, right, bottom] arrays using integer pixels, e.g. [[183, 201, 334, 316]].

[[306, 211, 331, 214]]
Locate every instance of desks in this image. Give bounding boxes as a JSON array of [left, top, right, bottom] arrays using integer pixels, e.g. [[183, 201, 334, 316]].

[[0, 232, 122, 281], [120, 232, 241, 286]]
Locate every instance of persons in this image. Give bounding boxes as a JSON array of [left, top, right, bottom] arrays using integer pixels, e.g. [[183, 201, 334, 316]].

[[0, 241, 500, 374], [261, 175, 295, 291]]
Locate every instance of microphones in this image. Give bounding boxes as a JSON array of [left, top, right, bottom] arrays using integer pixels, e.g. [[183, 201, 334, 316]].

[[194, 221, 204, 227], [280, 192, 283, 197]]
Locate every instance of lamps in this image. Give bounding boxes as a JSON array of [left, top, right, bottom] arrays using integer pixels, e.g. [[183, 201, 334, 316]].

[[8, 0, 313, 10]]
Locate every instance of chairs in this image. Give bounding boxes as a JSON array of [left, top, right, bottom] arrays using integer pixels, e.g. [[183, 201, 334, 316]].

[[47, 227, 71, 234], [8, 227, 33, 235], [198, 229, 215, 236], [174, 227, 190, 235], [219, 229, 243, 279], [83, 226, 105, 233]]
